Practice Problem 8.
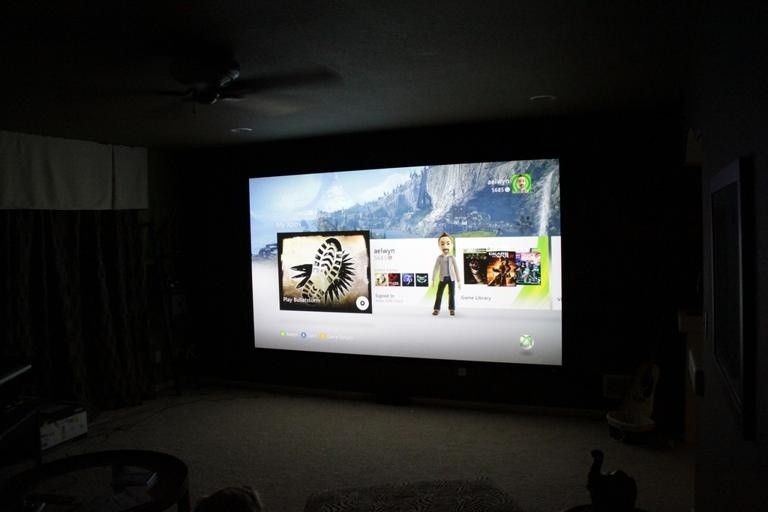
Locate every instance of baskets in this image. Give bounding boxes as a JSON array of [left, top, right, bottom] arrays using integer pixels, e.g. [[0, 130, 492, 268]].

[[606, 410, 653, 446]]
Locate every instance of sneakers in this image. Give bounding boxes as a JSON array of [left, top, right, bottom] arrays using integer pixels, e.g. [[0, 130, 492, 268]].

[[432, 310, 455, 315]]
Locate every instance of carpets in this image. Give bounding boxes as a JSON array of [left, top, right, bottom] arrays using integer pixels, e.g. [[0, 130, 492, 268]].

[[306, 476, 521, 511]]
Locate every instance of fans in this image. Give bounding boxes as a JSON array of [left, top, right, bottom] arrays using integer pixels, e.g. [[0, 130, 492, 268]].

[[118, 66, 345, 120]]
